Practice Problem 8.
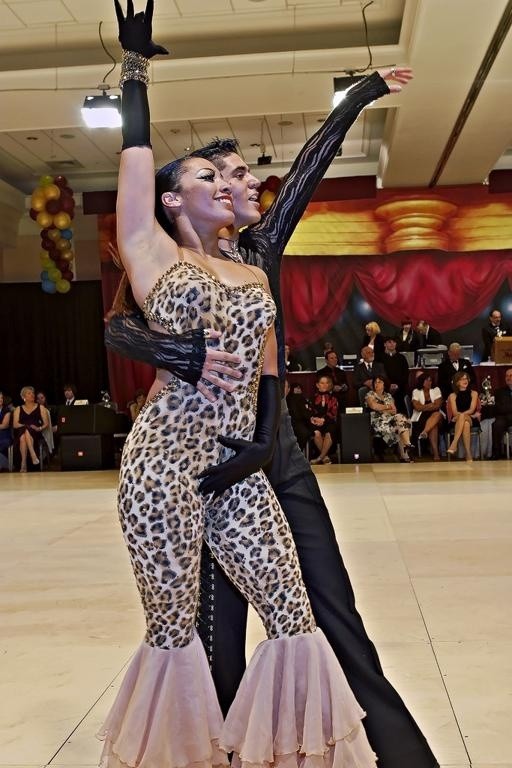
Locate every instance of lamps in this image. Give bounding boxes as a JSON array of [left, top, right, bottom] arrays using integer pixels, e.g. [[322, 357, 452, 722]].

[[80, 19, 123, 131], [256, 116, 272, 165], [329, 1, 376, 113]]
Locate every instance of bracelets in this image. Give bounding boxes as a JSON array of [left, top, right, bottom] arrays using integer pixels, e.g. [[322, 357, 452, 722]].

[[118, 49, 149, 88]]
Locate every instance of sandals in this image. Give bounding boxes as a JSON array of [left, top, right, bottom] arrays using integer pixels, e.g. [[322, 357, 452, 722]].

[[399, 456, 415, 463], [310, 456, 332, 465]]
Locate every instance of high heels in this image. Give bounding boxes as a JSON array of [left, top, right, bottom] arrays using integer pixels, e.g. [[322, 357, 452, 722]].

[[403, 443, 415, 453], [418, 432, 428, 440]]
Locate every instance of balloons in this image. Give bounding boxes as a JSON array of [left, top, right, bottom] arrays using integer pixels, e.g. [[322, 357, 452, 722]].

[[27, 173, 78, 296], [267, 174, 280, 193], [260, 189, 277, 211]]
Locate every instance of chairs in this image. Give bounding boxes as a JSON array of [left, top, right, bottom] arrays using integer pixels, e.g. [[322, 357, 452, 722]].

[[294, 386, 512, 464], [1, 405, 51, 471]]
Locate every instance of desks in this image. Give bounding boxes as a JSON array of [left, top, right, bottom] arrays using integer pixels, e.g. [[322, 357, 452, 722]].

[[286, 362, 512, 416]]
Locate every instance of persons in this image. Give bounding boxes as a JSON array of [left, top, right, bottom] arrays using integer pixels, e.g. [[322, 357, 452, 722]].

[[283, 307, 512, 463], [104, 65, 442, 768], [128, 388, 146, 424], [0, 381, 76, 473], [99, 388, 119, 414], [97, 0, 379, 768]]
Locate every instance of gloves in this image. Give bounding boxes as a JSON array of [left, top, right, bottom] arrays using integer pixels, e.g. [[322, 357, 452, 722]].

[[114, 0, 169, 150], [197, 374, 281, 499]]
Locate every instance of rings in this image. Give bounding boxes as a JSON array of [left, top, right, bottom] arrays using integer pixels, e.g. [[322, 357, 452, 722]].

[[389, 67, 398, 76]]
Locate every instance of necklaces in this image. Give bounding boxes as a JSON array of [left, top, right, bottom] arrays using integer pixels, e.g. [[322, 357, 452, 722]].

[[218, 234, 240, 259]]
